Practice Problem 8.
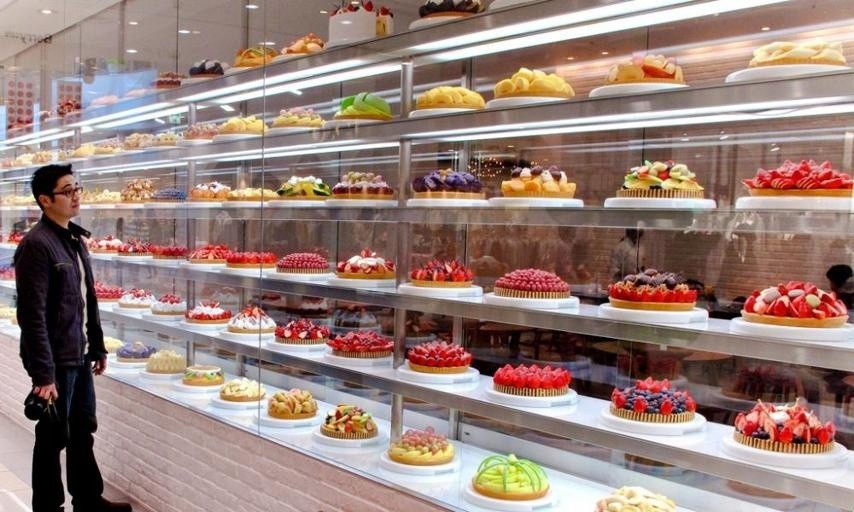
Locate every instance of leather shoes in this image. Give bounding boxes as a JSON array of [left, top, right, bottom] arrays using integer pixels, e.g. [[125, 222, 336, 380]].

[[71, 498, 131, 511]]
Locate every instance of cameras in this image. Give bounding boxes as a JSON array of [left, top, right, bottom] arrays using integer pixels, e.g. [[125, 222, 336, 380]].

[[24, 381, 59, 421]]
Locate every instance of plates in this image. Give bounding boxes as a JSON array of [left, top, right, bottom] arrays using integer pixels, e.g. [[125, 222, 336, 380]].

[[396, 365, 480, 384], [326, 279, 397, 288], [182, 202, 222, 208], [325, 199, 400, 208], [407, 198, 488, 208], [220, 268, 277, 276], [484, 386, 580, 408], [262, 129, 325, 136], [108, 358, 146, 368], [253, 409, 327, 428], [267, 199, 325, 208], [219, 328, 274, 341], [486, 97, 573, 111], [603, 197, 716, 209], [266, 338, 330, 353], [223, 66, 251, 77], [110, 254, 155, 263], [735, 196, 854, 212], [266, 272, 335, 282], [179, 320, 231, 330], [111, 304, 150, 314], [324, 119, 386, 128], [463, 486, 560, 512], [179, 77, 213, 88], [143, 202, 181, 207], [483, 292, 579, 307], [142, 312, 186, 321], [488, 197, 585, 208], [379, 451, 463, 474], [152, 259, 187, 264], [311, 430, 386, 447], [89, 204, 113, 210], [409, 16, 462, 28], [323, 352, 397, 367], [488, 0, 545, 12], [725, 63, 854, 82], [176, 140, 212, 145], [720, 431, 850, 470], [88, 251, 118, 261], [597, 302, 710, 325], [115, 203, 143, 208], [589, 83, 691, 97], [598, 406, 707, 436], [398, 282, 483, 296], [173, 379, 224, 393], [221, 201, 268, 208], [212, 134, 262, 144], [730, 316, 854, 342], [180, 261, 227, 270], [211, 394, 267, 409], [140, 367, 184, 380], [408, 108, 482, 120], [271, 53, 308, 63], [323, 40, 356, 49]]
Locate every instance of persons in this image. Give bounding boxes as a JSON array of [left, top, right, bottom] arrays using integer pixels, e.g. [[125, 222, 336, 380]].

[[610, 226, 644, 290], [16, 165, 134, 512], [824, 263, 853, 315]]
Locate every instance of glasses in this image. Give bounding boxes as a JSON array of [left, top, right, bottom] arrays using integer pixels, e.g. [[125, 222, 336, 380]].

[[46, 184, 83, 198]]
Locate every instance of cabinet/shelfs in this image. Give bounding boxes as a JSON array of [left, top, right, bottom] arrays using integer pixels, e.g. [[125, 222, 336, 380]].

[[0, 0, 854, 512]]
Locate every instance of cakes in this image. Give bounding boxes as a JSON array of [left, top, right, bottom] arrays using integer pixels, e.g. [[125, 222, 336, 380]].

[[268, 388, 317, 419], [118, 239, 153, 256], [271, 175, 330, 200], [217, 115, 269, 134], [330, 308, 381, 337], [605, 54, 684, 84], [3, 144, 117, 166], [466, 251, 497, 278], [121, 179, 154, 203], [713, 366, 807, 411], [94, 235, 122, 253], [8, 100, 83, 128], [226, 251, 276, 268], [0, 228, 27, 244], [735, 398, 836, 453], [116, 342, 156, 363], [151, 294, 188, 314], [519, 338, 590, 370], [298, 301, 328, 318], [611, 377, 695, 423], [233, 46, 278, 67], [494, 68, 575, 97], [93, 189, 122, 204], [472, 454, 549, 500], [147, 350, 184, 373], [335, 247, 396, 279], [183, 365, 225, 386], [688, 279, 721, 312], [329, 0, 395, 41], [189, 245, 231, 263], [78, 191, 93, 204], [388, 426, 453, 466], [220, 378, 265, 401], [274, 318, 330, 344], [152, 72, 187, 88], [321, 404, 378, 439], [89, 95, 118, 106], [493, 364, 571, 396], [228, 304, 277, 333], [593, 486, 677, 512], [185, 302, 233, 324], [494, 269, 571, 298], [332, 172, 394, 199], [184, 123, 218, 139], [408, 341, 472, 374], [741, 159, 854, 197], [150, 189, 188, 203], [741, 280, 849, 328], [335, 92, 392, 120], [410, 259, 473, 288], [191, 182, 232, 202], [281, 33, 327, 54], [153, 246, 189, 259], [415, 86, 485, 108], [81, 235, 94, 250], [326, 330, 393, 358], [614, 355, 689, 389], [0, 195, 39, 206], [608, 269, 698, 311], [727, 479, 794, 499], [419, 0, 485, 18], [151, 133, 176, 146], [124, 133, 154, 148], [501, 165, 576, 198], [215, 286, 252, 305], [118, 288, 156, 308], [564, 264, 602, 295], [0, 263, 15, 281], [126, 89, 148, 96], [405, 320, 436, 348], [103, 336, 124, 354], [615, 160, 704, 198], [0, 303, 18, 325], [412, 168, 486, 199], [226, 187, 273, 201], [271, 107, 323, 128], [749, 42, 849, 67], [94, 281, 127, 302], [252, 293, 286, 310], [277, 253, 329, 274], [189, 59, 230, 77]]
